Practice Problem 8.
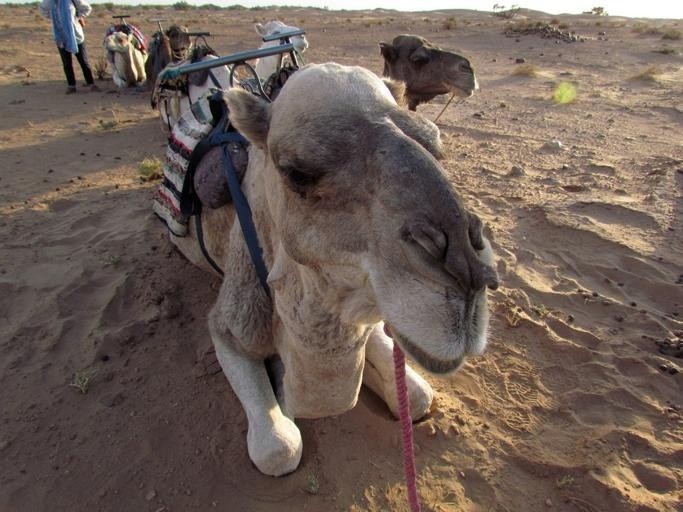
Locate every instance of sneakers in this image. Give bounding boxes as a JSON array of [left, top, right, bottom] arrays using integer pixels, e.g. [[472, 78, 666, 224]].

[[65, 86, 77, 94], [91, 85, 101, 92]]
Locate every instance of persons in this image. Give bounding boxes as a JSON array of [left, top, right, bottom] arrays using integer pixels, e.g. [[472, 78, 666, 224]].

[[39, 0, 99, 95]]
